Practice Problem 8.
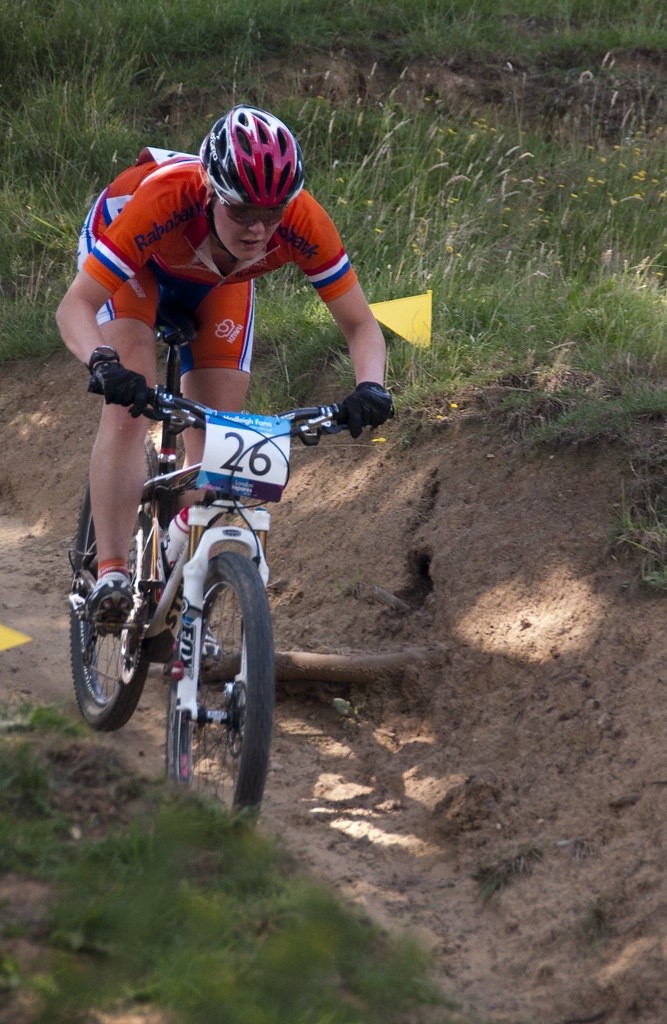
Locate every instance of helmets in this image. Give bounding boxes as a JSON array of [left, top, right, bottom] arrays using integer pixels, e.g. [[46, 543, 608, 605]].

[[199, 103, 306, 208]]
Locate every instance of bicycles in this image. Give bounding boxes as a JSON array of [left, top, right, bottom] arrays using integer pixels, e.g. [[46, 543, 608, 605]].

[[70, 376, 393, 843]]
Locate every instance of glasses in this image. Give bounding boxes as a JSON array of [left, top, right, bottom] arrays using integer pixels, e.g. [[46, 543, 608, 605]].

[[212, 184, 288, 226]]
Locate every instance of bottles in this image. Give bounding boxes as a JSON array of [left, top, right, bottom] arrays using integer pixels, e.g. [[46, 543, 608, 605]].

[[157, 508, 195, 568]]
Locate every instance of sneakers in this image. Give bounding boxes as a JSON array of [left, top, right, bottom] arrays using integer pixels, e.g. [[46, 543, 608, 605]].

[[202, 623, 221, 680], [85, 572, 134, 638]]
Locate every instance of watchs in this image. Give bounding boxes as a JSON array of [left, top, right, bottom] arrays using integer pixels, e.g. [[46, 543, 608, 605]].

[[84, 343, 121, 374]]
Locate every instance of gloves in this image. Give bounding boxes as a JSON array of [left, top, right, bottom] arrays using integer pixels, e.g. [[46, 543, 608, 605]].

[[93, 361, 147, 418], [336, 381, 393, 439]]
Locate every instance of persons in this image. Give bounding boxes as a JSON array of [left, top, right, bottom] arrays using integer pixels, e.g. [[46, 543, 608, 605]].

[[56, 101, 389, 666]]
